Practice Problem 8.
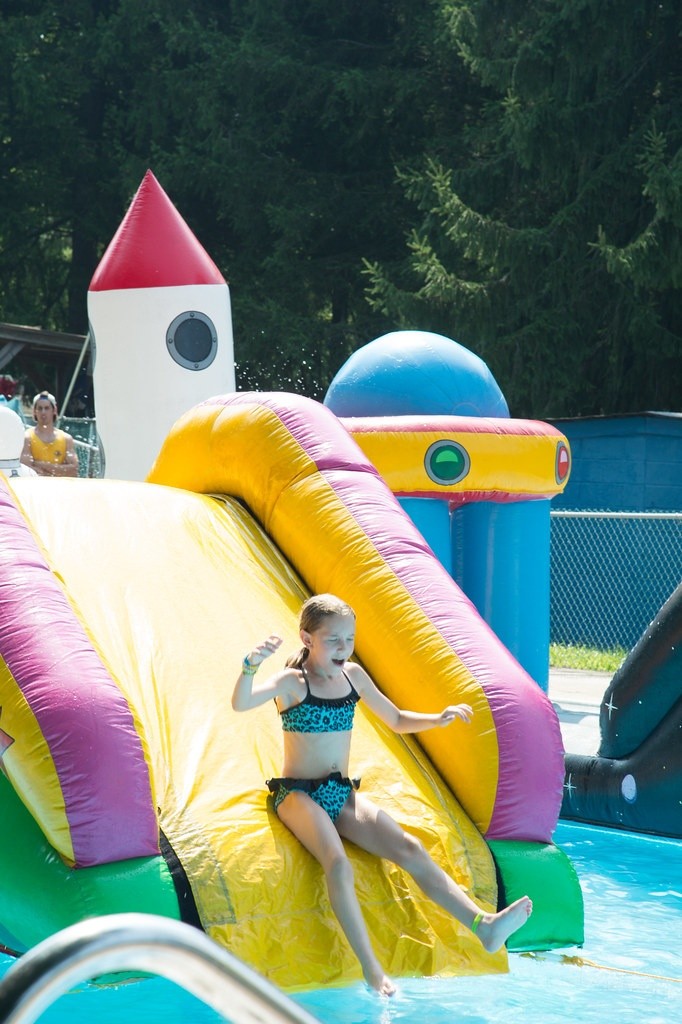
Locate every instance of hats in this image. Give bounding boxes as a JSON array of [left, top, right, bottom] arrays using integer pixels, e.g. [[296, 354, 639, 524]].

[[32, 392, 58, 424]]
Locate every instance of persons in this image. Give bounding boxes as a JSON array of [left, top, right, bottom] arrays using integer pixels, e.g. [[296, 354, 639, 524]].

[[16, 391, 79, 478], [232, 594, 534, 1000]]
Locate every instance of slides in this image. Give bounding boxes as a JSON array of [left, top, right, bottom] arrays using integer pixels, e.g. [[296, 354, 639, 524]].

[[1, 385, 591, 990]]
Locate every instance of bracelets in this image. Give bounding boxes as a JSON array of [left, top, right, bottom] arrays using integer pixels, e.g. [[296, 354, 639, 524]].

[[241, 656, 258, 676]]
